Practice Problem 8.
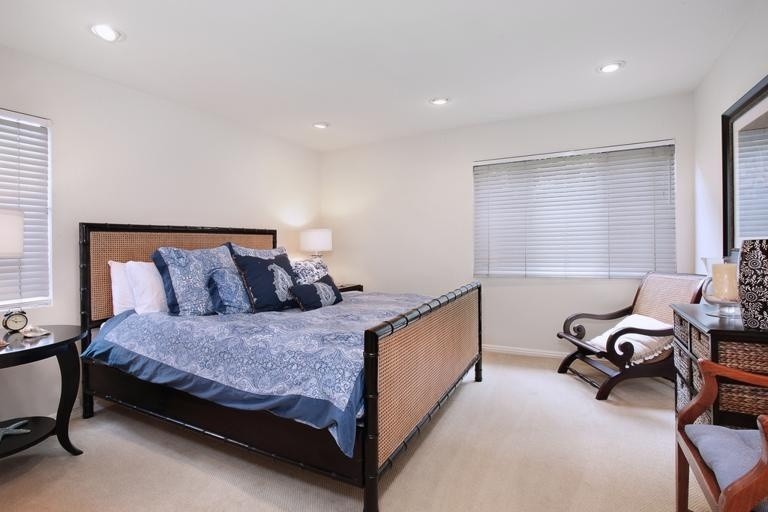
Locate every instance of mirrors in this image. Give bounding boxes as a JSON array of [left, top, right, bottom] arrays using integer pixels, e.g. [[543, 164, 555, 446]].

[[721, 75, 768, 257]]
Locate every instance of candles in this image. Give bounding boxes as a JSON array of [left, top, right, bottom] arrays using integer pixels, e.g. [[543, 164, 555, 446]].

[[712, 263, 736, 301]]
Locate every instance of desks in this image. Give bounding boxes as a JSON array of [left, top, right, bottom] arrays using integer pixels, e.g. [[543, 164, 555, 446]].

[[0, 324, 89, 458]]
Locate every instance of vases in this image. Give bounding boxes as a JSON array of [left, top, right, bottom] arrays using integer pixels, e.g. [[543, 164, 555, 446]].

[[738, 239, 768, 331]]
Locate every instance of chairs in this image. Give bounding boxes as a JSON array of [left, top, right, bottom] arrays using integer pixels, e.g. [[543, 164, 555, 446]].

[[557, 271, 707, 401], [673, 357, 768, 511]]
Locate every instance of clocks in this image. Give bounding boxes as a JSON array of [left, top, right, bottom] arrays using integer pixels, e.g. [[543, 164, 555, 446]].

[[2, 308, 28, 335]]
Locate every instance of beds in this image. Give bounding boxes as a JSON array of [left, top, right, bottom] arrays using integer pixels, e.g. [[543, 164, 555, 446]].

[[79, 221, 483, 512]]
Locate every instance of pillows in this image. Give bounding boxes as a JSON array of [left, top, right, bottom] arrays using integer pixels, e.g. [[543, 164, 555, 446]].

[[108, 260, 135, 316], [290, 274, 343, 312], [291, 255, 330, 284], [232, 253, 299, 313], [126, 260, 169, 315], [152, 244, 239, 317], [224, 242, 285, 259], [206, 266, 253, 315], [586, 314, 674, 368]]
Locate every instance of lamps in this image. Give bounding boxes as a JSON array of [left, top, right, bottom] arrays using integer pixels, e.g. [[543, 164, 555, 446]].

[[299, 229, 333, 258]]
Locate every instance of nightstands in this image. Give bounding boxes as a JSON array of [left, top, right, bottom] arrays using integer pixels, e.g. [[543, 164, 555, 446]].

[[338, 283, 363, 292]]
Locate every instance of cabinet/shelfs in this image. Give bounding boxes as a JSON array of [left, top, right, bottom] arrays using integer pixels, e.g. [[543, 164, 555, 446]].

[[668, 303, 768, 430]]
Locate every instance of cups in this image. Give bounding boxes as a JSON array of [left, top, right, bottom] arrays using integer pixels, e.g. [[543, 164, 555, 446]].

[[698, 256, 741, 319]]
